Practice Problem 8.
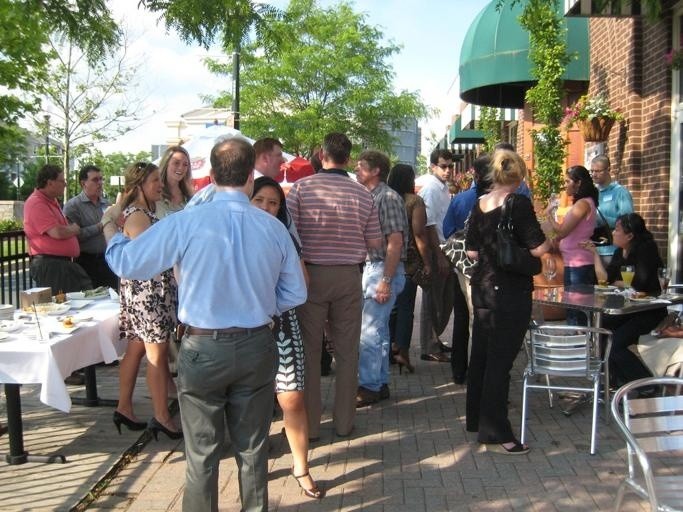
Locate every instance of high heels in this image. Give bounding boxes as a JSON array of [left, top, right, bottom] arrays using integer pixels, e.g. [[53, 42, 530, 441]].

[[480, 439, 530, 459], [397, 354, 417, 375], [149, 417, 184, 442], [290, 465, 322, 497], [113, 410, 148, 435]]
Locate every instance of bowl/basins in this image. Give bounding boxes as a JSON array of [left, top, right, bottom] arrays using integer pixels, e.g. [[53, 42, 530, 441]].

[[65, 292, 85, 300]]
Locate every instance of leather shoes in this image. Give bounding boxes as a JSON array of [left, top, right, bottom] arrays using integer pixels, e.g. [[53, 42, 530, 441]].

[[420, 352, 452, 362], [379, 383, 390, 401], [440, 342, 452, 353], [354, 386, 381, 408]]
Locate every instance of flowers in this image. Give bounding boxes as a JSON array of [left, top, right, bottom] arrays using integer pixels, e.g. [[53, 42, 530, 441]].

[[560, 89, 627, 129]]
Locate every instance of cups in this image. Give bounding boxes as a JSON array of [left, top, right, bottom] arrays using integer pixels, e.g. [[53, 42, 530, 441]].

[[656, 266, 672, 299], [620, 265, 635, 309], [542, 258, 558, 297], [36, 325, 51, 344]]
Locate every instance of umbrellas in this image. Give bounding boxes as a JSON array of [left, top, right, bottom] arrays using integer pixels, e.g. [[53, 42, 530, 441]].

[[153, 121, 315, 195]]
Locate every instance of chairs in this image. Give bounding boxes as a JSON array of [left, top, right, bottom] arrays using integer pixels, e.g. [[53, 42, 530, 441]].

[[609, 375, 683, 508], [518, 323, 614, 457]]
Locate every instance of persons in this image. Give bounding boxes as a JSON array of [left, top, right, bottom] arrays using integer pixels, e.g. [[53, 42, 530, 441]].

[[441, 159, 493, 237], [420, 150, 457, 364], [546, 166, 599, 326], [102, 138, 307, 511], [387, 165, 432, 375], [467, 151, 548, 455], [251, 137, 311, 295], [591, 153, 635, 264], [469, 142, 530, 200], [285, 133, 385, 436], [353, 150, 406, 405], [63, 165, 120, 289], [252, 176, 322, 498], [113, 162, 184, 442], [22, 165, 120, 384], [579, 213, 663, 397], [160, 148, 191, 219]]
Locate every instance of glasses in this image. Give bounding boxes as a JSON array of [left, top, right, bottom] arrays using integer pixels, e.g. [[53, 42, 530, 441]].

[[85, 177, 103, 185], [135, 161, 146, 178], [435, 163, 454, 169], [590, 168, 607, 173], [51, 178, 67, 188]]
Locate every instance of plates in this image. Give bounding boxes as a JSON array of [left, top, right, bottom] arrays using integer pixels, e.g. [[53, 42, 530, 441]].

[[628, 296, 656, 302], [62, 298, 96, 309], [87, 286, 109, 300], [593, 283, 620, 291], [21, 303, 72, 316], [50, 324, 82, 335]]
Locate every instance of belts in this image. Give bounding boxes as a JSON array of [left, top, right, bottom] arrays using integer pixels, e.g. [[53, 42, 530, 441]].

[[175, 323, 268, 340], [29, 253, 78, 263]]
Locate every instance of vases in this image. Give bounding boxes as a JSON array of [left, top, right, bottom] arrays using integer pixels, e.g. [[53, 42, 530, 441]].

[[576, 118, 615, 143]]
